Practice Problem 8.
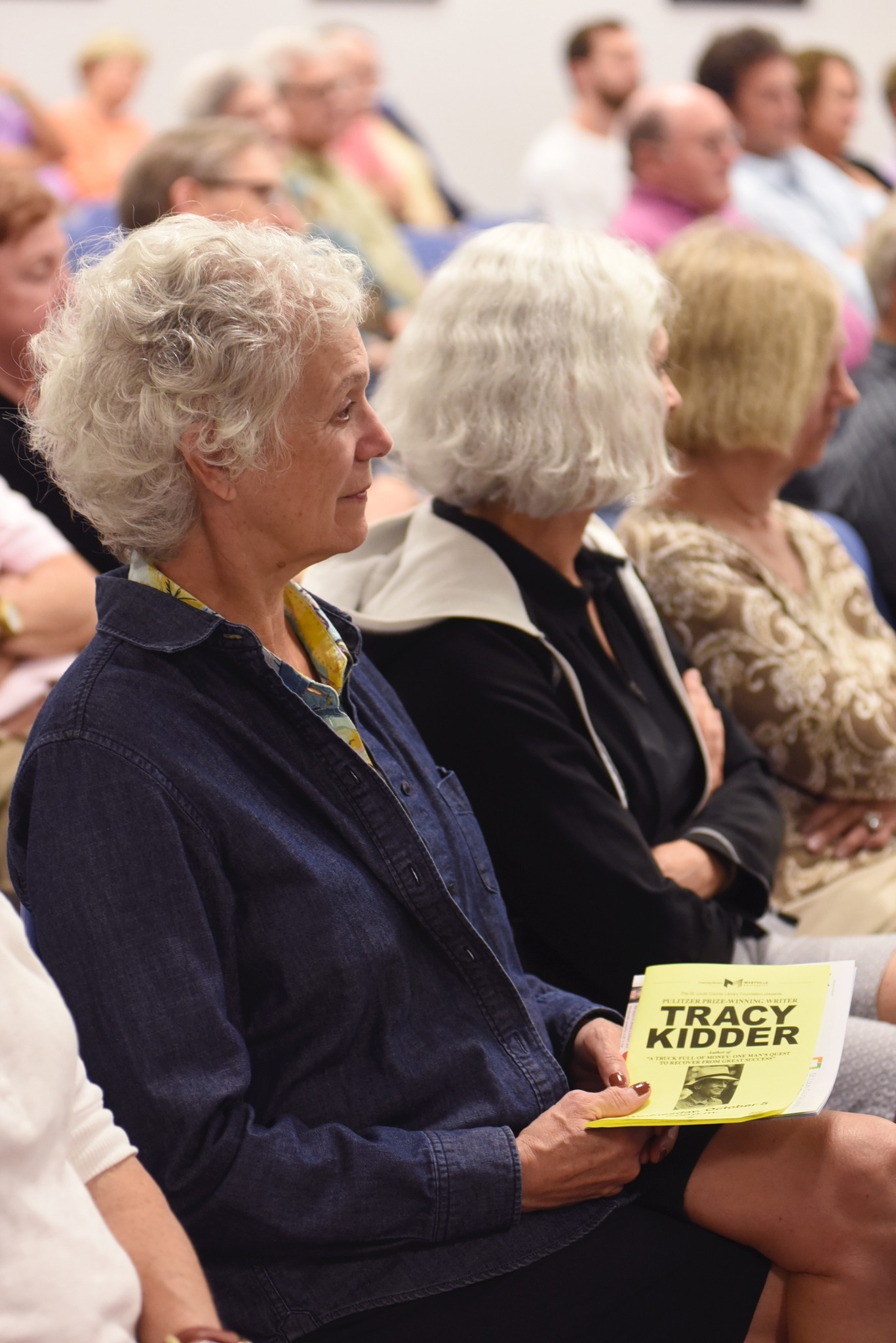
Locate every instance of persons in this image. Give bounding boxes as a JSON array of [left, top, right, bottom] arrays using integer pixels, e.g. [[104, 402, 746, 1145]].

[[783, 42, 896, 207], [0, 28, 476, 399], [598, 215, 896, 940], [602, 78, 872, 372], [0, 878, 252, 1343], [690, 17, 895, 261], [14, 214, 896, 1343], [801, 215, 896, 627], [333, 215, 896, 1128], [0, 467, 117, 879], [500, 10, 652, 223], [0, 150, 138, 576]]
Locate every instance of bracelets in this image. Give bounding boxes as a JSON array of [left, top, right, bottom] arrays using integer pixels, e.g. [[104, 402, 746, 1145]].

[[163, 1326, 242, 1343]]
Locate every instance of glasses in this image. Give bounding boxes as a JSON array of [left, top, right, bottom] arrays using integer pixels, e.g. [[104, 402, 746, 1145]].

[[205, 177, 273, 201]]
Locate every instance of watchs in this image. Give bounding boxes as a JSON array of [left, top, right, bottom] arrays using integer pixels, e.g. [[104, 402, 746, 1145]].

[[0, 603, 25, 656]]
